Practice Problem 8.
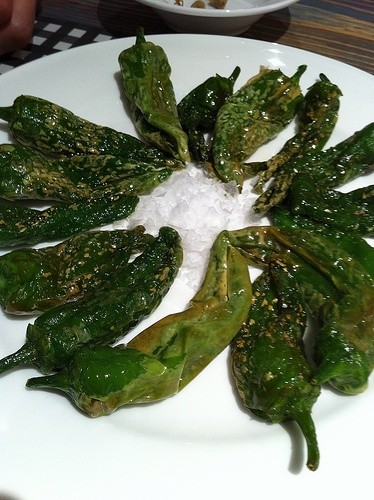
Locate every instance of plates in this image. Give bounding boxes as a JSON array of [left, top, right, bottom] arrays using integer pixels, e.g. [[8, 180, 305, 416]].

[[134, 0, 301, 37], [0, 30, 373, 499]]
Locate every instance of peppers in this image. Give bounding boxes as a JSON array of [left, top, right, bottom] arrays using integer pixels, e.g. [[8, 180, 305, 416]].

[[0, 19, 374, 472]]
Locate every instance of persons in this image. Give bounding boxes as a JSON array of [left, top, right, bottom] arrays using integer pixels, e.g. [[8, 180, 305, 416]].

[[0, 0, 37, 58]]
